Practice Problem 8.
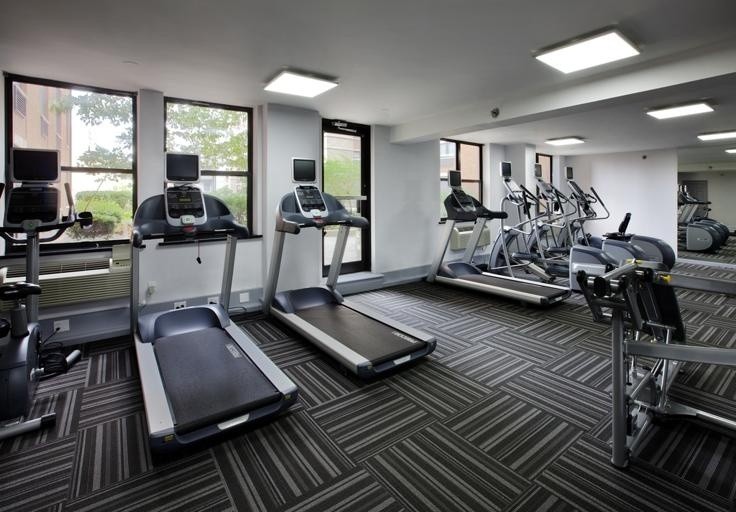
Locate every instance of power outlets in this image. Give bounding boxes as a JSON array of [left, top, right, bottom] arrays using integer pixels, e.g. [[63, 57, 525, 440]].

[[207, 297, 221, 305], [53, 319, 71, 332], [175, 300, 188, 311]]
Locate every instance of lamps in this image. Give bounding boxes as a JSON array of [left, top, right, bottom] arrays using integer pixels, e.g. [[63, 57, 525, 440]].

[[544, 137, 586, 147], [646, 103, 736, 155], [263, 70, 339, 99], [535, 27, 639, 75]]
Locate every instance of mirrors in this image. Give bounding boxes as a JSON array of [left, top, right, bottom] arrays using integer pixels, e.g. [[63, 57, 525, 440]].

[[676, 148, 736, 268]]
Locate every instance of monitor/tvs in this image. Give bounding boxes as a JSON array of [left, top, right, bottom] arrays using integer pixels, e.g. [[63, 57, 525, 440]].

[[684, 184, 687, 192], [535, 163, 542, 177], [500, 161, 512, 177], [165, 152, 201, 184], [10, 148, 61, 183], [565, 166, 573, 179], [448, 170, 462, 187], [679, 184, 683, 192], [292, 158, 317, 183]]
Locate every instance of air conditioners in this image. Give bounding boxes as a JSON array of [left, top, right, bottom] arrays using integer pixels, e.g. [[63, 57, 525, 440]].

[[451, 223, 491, 250], [1, 244, 132, 312]]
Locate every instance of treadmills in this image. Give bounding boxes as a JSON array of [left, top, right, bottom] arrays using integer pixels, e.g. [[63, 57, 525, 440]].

[[263, 157, 436, 377], [426, 170, 571, 305], [131, 152, 299, 467]]
